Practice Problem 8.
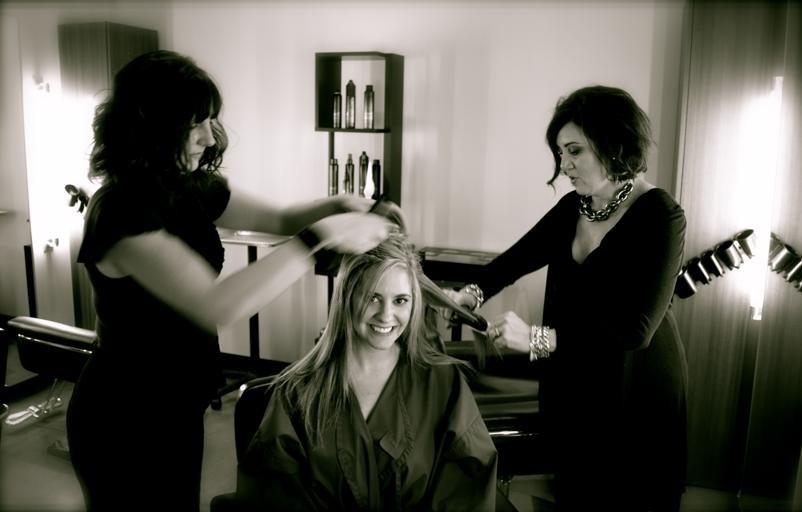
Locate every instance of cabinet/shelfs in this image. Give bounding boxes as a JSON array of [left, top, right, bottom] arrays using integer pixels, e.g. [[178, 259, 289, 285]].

[[311, 49, 405, 276], [55, 19, 158, 100]]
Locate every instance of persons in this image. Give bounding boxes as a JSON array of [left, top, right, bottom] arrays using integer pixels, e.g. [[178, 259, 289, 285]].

[[435, 86, 689, 511], [248, 228, 499, 512], [66, 49, 409, 511]]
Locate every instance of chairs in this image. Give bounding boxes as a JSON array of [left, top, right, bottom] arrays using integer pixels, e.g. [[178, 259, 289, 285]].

[[3, 314, 99, 459], [208, 371, 525, 512]]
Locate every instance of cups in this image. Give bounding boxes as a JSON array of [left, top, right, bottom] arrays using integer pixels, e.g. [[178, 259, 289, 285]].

[[769, 235, 802, 294], [673, 230, 759, 300]]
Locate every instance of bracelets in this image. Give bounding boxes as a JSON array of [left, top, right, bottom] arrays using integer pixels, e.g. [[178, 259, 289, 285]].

[[463, 282, 485, 310], [529, 324, 551, 362], [297, 228, 328, 263], [369, 196, 383, 213]]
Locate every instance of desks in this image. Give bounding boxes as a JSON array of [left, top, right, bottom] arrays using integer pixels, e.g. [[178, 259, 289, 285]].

[[209, 242, 333, 366]]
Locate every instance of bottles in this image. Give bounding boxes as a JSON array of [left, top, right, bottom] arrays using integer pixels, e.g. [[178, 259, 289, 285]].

[[330, 152, 380, 201], [332, 78, 375, 130]]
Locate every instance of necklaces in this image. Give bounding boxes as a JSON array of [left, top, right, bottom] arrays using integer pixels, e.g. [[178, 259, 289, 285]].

[[578, 174, 636, 223]]
